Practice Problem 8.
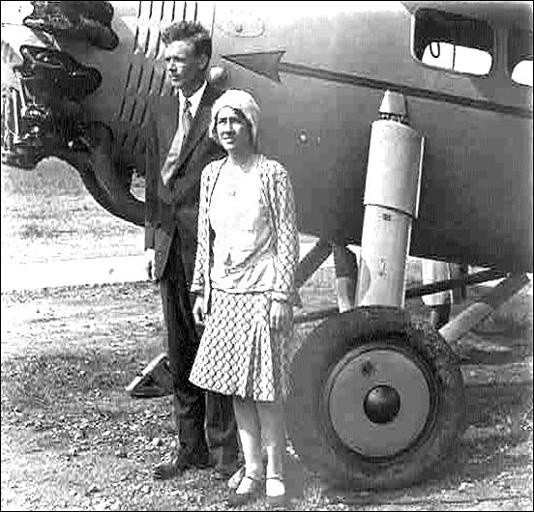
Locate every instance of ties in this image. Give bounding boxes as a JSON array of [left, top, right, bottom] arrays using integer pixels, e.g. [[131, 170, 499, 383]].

[[160, 99, 193, 188]]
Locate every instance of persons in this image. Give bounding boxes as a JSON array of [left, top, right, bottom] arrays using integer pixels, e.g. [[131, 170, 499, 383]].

[[190, 89, 304, 508], [144, 18, 229, 480]]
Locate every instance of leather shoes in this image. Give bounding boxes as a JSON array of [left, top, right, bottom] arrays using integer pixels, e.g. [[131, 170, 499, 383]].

[[151, 452, 230, 480]]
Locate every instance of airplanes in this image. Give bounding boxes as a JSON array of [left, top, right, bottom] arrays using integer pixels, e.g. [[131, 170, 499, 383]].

[[2, 0, 533, 494]]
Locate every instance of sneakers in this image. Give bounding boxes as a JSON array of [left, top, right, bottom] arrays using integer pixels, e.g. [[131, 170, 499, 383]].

[[265, 474, 288, 505], [230, 472, 263, 505]]
